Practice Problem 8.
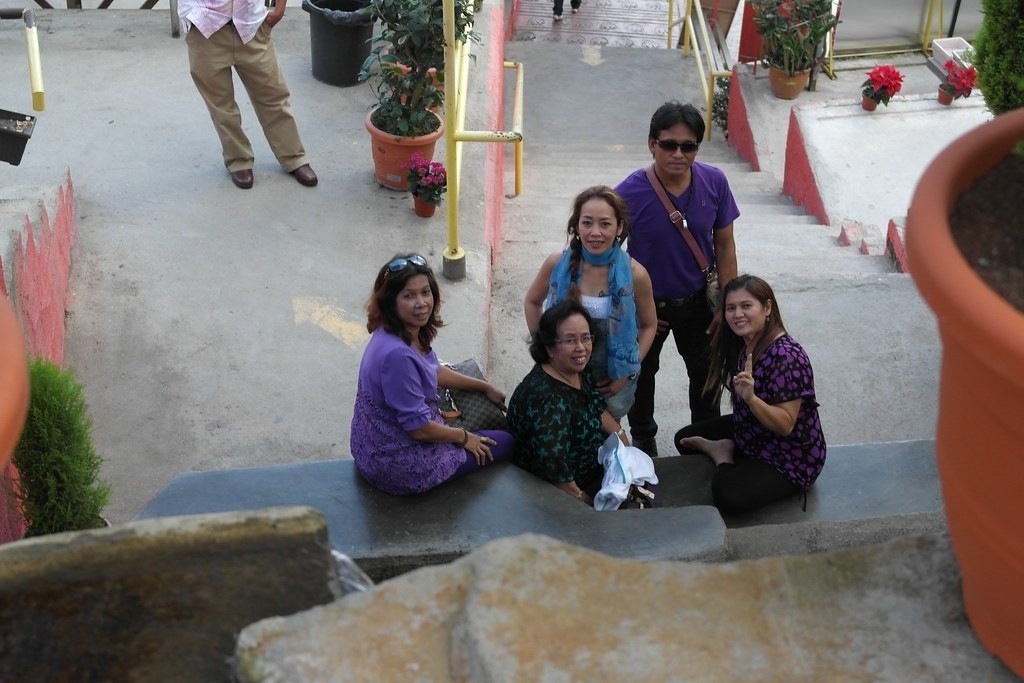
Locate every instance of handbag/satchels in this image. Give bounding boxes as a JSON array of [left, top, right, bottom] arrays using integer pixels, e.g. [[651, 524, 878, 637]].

[[436, 358, 509, 434], [705, 268, 722, 316]]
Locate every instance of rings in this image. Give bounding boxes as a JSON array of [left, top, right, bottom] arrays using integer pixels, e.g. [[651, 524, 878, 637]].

[[481, 437, 486, 442], [749, 374, 751, 378]]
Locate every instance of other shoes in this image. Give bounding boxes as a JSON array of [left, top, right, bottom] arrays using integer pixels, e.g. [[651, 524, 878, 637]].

[[572, 8, 578, 13], [554, 14, 563, 20]]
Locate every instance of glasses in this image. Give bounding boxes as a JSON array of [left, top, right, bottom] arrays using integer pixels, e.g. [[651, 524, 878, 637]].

[[383, 255, 428, 279], [554, 334, 595, 348], [655, 139, 698, 153]]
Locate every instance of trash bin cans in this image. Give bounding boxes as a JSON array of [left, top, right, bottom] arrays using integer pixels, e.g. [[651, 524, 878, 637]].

[[306, 0, 379, 88]]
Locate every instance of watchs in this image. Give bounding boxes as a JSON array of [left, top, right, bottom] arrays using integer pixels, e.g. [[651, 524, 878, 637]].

[[630, 374, 637, 380], [618, 427, 625, 435]]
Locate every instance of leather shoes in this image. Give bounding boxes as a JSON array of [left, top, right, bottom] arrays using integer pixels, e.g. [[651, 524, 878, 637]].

[[233, 168, 253, 188], [293, 162, 318, 185]]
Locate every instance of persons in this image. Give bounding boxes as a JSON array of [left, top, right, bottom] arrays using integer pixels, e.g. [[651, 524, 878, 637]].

[[176, 0, 318, 187], [523, 185, 657, 425], [674, 274, 827, 515], [613, 97, 740, 458], [552, 0, 582, 20], [350, 251, 515, 497], [505, 299, 634, 510]]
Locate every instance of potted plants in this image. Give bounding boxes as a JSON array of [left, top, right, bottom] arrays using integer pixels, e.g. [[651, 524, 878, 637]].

[[357, 0, 482, 192], [2, 351, 113, 541], [748, 0, 843, 99], [952, 49, 980, 74]]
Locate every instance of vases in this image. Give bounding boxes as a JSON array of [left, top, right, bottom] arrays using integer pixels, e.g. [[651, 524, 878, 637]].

[[905, 108, 1024, 681], [413, 195, 436, 218], [862, 90, 877, 111], [932, 38, 973, 69], [937, 84, 953, 105]]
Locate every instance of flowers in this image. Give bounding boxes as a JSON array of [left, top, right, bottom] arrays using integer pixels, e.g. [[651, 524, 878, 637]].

[[399, 152, 447, 208], [860, 63, 906, 108], [942, 59, 977, 102]]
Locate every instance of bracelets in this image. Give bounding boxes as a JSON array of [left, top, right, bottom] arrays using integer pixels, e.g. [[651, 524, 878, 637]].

[[577, 491, 583, 499], [452, 429, 468, 448]]
